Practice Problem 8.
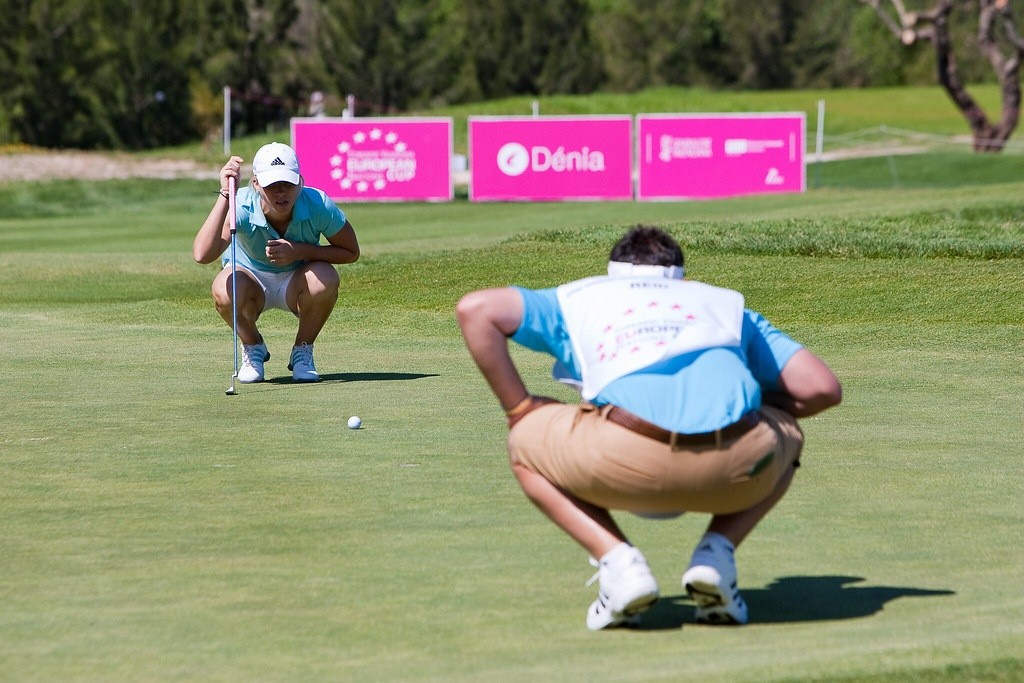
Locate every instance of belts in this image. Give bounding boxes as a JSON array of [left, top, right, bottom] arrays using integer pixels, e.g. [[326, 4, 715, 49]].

[[597, 402, 758, 448]]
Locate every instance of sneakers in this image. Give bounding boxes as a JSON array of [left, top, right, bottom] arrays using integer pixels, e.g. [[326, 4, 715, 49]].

[[237, 336, 270, 383], [586, 545, 659, 629], [286, 341, 319, 382], [681, 539, 748, 625]]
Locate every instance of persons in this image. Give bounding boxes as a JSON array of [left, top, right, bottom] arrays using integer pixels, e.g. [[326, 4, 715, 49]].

[[193, 142, 361, 384], [457, 223, 842, 631]]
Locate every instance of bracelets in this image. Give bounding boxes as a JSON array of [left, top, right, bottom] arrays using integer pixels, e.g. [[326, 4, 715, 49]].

[[507, 395, 532, 416], [213, 190, 237, 199]]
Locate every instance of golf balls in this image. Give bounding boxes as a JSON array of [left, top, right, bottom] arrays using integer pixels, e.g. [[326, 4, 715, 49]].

[[348, 416, 361, 429]]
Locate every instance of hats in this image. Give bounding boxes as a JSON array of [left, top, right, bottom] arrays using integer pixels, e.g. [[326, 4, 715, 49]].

[[252, 142, 301, 187]]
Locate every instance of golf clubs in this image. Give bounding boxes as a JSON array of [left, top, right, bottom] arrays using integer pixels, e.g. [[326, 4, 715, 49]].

[[224, 175, 240, 395]]
